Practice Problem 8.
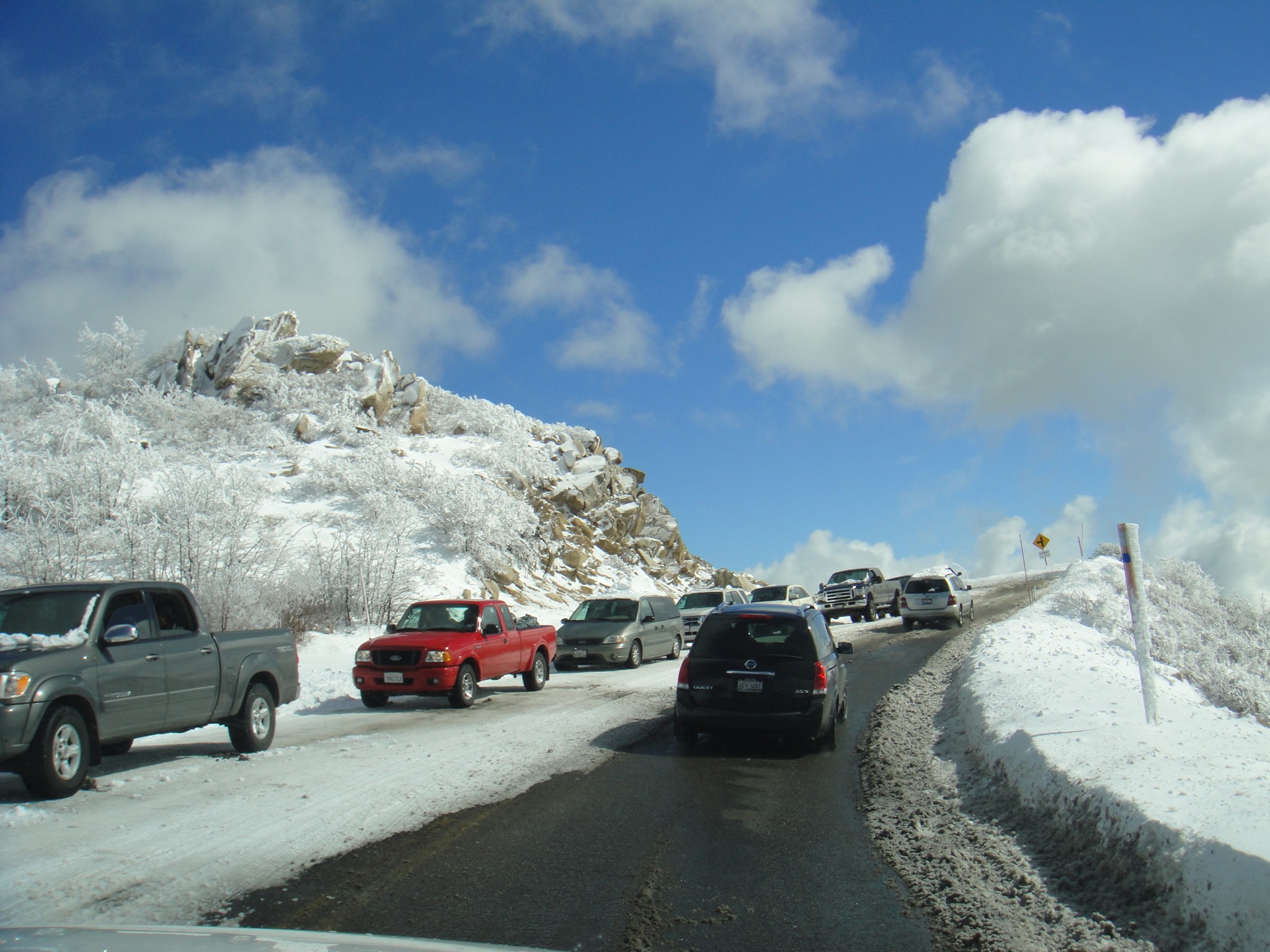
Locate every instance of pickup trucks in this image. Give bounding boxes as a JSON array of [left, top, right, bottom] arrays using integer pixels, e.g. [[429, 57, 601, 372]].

[[0, 581, 300, 799], [818, 567, 903, 625], [353, 600, 557, 707]]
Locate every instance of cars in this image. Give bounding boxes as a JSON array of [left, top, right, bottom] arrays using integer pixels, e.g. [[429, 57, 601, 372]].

[[747, 584, 814, 607]]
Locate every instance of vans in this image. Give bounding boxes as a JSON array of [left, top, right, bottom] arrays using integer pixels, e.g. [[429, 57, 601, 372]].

[[675, 588, 751, 644], [553, 593, 684, 671]]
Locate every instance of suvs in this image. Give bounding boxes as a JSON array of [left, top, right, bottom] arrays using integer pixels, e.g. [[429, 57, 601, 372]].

[[901, 570, 974, 629], [674, 602, 853, 753]]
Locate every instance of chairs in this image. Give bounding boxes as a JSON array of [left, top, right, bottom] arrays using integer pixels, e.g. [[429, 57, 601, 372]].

[[587, 605, 610, 619], [426, 608, 451, 628]]
